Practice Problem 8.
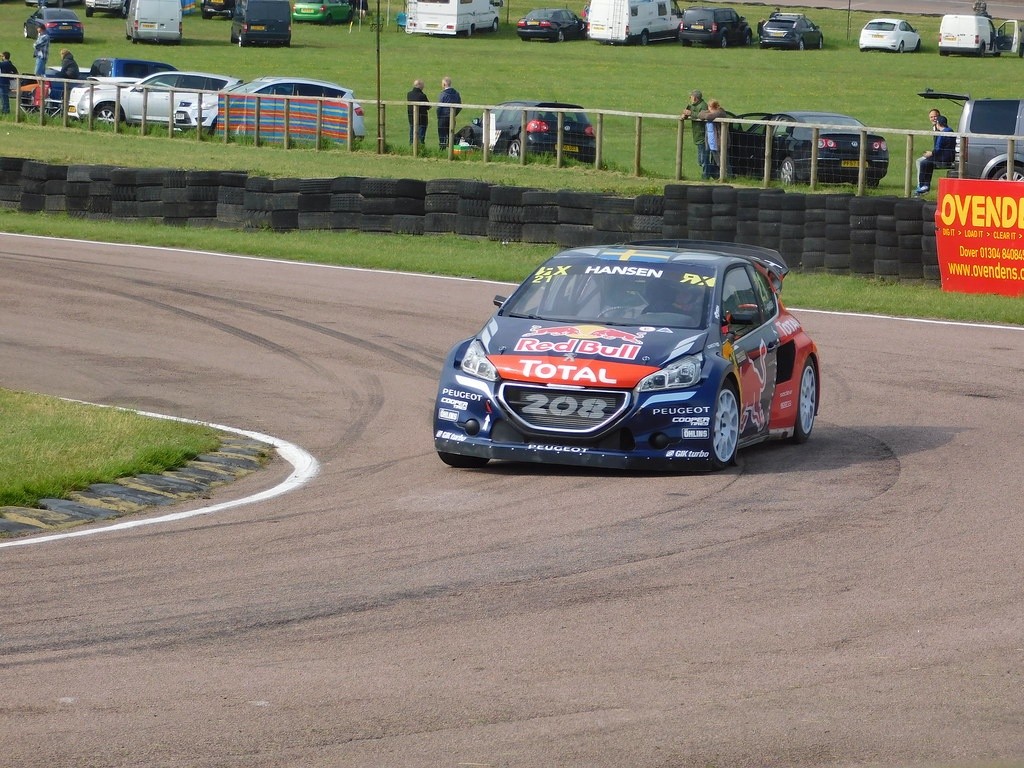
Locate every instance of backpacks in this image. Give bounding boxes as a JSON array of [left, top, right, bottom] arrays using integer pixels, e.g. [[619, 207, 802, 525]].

[[454, 126, 474, 146]]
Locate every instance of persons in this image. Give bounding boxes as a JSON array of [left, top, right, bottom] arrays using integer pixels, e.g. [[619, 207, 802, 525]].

[[681, 91, 711, 181], [669, 287, 701, 321], [699, 100, 736, 180], [769, 7, 780, 19], [0, 52, 17, 112], [913, 116, 956, 194], [1020, 25, 1024, 57], [55, 49, 79, 80], [757, 19, 766, 38], [916, 109, 940, 189], [33, 24, 50, 76], [407, 80, 432, 145], [437, 77, 462, 150]]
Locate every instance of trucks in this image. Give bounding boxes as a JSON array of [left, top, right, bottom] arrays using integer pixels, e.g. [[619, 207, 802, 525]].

[[586, 0, 686, 46], [405, 0, 503, 37]]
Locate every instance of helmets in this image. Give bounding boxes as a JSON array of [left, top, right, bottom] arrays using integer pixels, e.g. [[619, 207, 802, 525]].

[[670, 282, 703, 313]]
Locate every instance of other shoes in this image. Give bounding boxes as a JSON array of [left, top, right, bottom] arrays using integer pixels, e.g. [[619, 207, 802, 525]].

[[916, 185, 930, 192]]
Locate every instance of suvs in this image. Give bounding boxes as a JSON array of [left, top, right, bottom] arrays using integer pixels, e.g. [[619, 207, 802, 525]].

[[293, 0, 354, 26], [678, 6, 752, 48], [85, 0, 129, 17], [173, 76, 365, 143], [758, 12, 823, 50], [915, 88, 1024, 182], [66, 71, 244, 128]]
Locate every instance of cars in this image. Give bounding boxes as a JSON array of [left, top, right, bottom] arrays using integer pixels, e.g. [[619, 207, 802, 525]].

[[20, 64, 90, 113], [707, 109, 889, 189], [859, 18, 921, 53], [23, 8, 84, 43], [432, 240, 821, 469], [200, 0, 237, 19], [517, 8, 587, 43], [454, 101, 598, 165]]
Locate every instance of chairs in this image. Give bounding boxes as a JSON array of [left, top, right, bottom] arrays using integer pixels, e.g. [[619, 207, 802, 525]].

[[648, 277, 676, 306], [786, 125, 795, 136], [370, 16, 383, 31], [397, 11, 406, 32], [538, 112, 572, 120], [19, 80, 65, 118], [577, 271, 626, 317]]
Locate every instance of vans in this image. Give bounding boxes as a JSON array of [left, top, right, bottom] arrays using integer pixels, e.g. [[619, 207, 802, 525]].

[[90, 58, 179, 78], [938, 14, 1019, 58], [126, 0, 183, 45], [230, 0, 292, 48]]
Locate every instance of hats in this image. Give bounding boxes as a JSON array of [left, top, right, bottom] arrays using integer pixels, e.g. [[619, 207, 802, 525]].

[[35, 23, 46, 32]]
